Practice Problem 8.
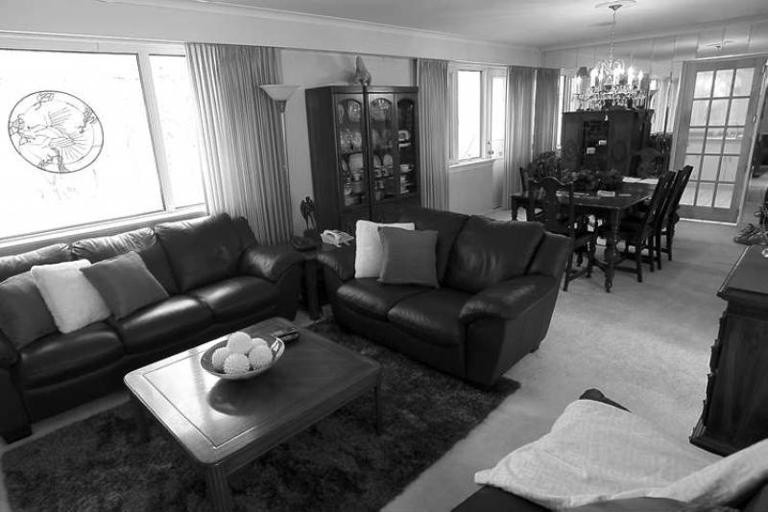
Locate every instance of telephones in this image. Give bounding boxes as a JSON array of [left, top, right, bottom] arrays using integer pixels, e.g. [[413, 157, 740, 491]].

[[290, 235, 317, 251], [320, 229, 355, 248]]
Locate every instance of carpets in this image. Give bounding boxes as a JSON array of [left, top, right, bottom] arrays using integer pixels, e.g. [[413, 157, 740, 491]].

[[0, 316, 522, 511]]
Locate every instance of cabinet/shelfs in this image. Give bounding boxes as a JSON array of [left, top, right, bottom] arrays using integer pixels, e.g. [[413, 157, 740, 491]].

[[305, 84, 422, 233], [688, 244, 768, 458], [560, 108, 655, 177]]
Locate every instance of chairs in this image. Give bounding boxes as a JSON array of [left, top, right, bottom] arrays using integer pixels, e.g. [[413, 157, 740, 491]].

[[510, 150, 693, 293]]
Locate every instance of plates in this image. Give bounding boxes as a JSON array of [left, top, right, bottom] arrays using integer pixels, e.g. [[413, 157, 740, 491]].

[[383, 153, 393, 166], [373, 155, 382, 168], [200, 333, 285, 380]]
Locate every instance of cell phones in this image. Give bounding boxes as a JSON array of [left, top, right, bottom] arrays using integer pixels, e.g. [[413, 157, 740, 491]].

[[271, 326, 297, 338], [279, 332, 299, 343]]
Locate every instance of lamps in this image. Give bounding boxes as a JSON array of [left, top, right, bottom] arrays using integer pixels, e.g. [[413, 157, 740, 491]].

[[571, 4, 659, 112], [258, 83, 301, 241]]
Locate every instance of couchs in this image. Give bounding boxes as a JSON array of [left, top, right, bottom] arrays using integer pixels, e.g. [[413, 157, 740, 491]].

[[0, 212, 306, 445], [315, 202, 574, 391], [448, 388, 768, 510]]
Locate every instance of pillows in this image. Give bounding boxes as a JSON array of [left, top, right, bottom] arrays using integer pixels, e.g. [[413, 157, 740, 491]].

[[354, 205, 545, 294], [0, 212, 245, 351]]
